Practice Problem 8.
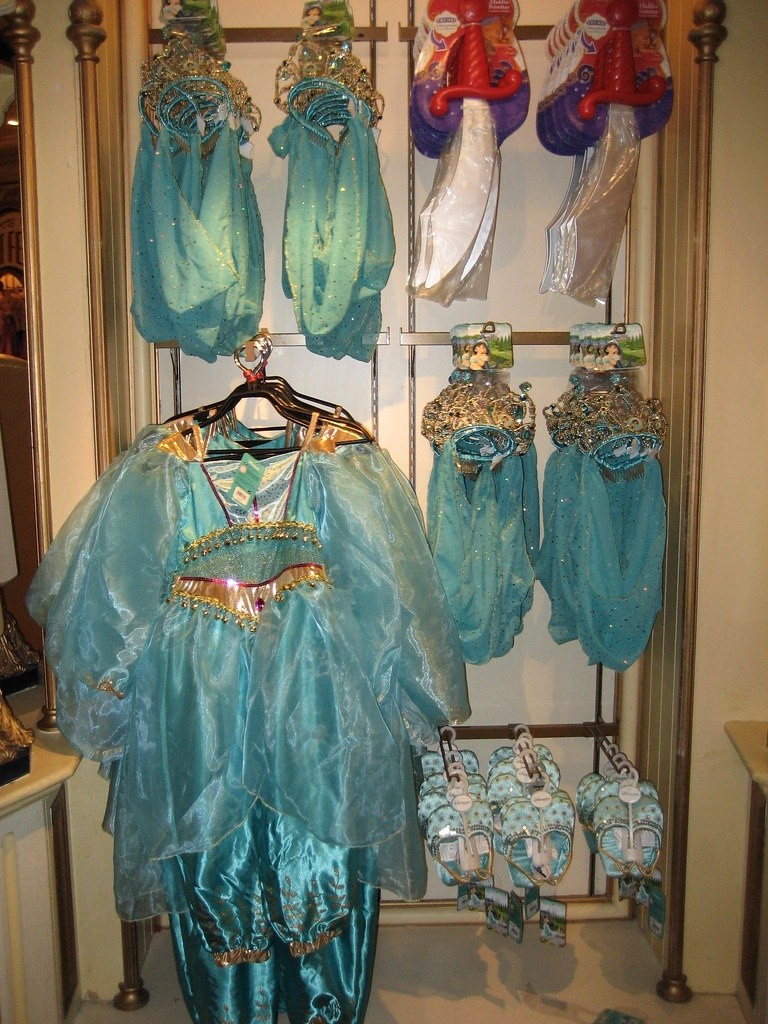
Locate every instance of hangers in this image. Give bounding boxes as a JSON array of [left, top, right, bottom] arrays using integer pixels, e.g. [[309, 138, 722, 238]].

[[157, 334, 372, 464]]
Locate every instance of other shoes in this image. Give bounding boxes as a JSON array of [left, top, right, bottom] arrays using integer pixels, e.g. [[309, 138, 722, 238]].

[[417, 743, 576, 887], [576, 773, 663, 878]]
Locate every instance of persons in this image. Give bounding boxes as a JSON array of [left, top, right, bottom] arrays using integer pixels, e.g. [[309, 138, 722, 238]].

[[571, 340, 623, 371], [486, 902, 498, 931], [456, 337, 493, 373], [637, 878, 650, 906], [540, 914, 553, 942], [466, 883, 483, 910]]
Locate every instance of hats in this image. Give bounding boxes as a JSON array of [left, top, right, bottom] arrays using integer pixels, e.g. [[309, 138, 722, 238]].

[[311, 3, 322, 9], [476, 338, 489, 347], [605, 339, 620, 347]]
